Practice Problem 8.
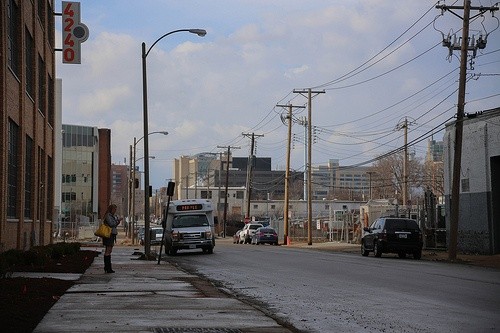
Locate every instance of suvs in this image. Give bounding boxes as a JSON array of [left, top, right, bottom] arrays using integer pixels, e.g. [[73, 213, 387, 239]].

[[360, 217, 424, 260]]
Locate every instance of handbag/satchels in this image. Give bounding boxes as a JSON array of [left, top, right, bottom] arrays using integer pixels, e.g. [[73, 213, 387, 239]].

[[94, 215, 113, 238]]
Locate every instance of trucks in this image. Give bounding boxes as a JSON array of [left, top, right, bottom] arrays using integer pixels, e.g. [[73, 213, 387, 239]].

[[161, 198, 215, 255]]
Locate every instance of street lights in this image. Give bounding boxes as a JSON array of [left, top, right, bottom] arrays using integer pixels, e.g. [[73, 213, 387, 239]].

[[127, 131, 169, 246], [128, 144, 155, 238], [141, 28, 207, 258]]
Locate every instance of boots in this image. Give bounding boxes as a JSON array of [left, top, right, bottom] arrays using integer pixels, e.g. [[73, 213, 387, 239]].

[[103, 254, 115, 273]]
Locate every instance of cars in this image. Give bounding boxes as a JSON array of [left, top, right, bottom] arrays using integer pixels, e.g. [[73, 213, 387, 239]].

[[133, 224, 163, 246], [250, 227, 278, 246], [233, 230, 243, 244], [254, 213, 327, 230]]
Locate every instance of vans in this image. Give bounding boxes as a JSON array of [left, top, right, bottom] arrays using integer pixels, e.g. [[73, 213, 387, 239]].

[[238, 223, 264, 244]]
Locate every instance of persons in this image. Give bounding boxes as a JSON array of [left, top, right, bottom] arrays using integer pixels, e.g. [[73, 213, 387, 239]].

[[103, 204, 124, 273]]
